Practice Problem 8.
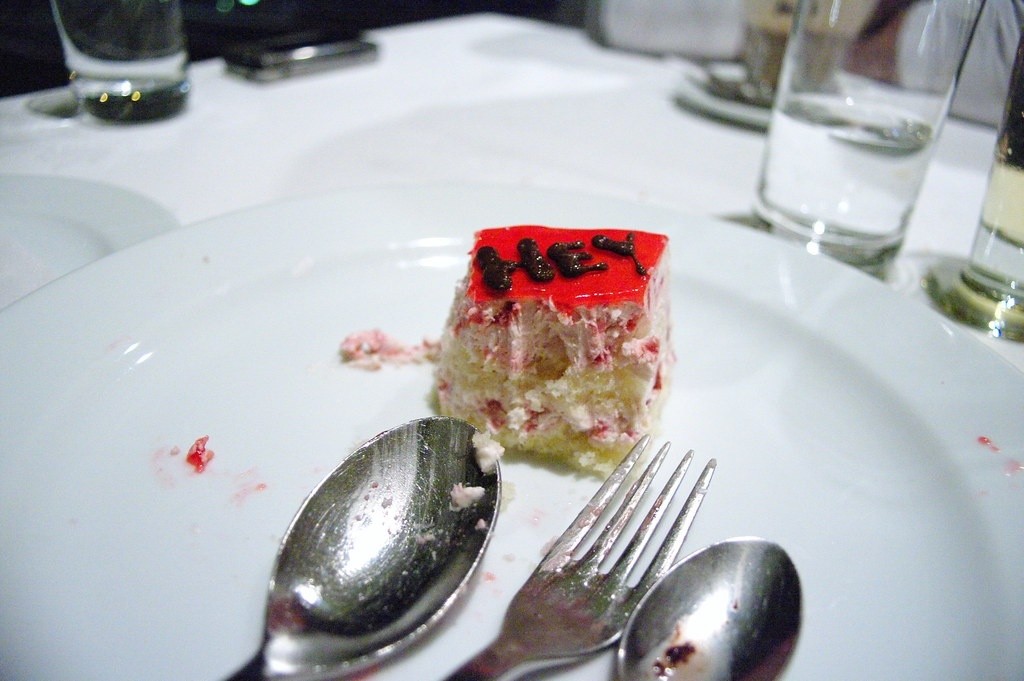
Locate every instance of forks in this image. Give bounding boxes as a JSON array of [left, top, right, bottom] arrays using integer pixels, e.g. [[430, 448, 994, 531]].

[[441, 434, 716, 681]]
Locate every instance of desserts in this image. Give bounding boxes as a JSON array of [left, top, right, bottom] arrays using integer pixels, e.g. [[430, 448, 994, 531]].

[[436, 223, 673, 481], [740, 0, 846, 102]]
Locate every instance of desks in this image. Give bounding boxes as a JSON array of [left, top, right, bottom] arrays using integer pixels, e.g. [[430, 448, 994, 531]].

[[0, 10, 1024, 364]]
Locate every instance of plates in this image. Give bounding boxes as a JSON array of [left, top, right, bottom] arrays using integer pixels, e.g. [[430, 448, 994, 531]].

[[0, 175, 182, 312], [0, 180, 1024, 681], [675, 67, 772, 129]]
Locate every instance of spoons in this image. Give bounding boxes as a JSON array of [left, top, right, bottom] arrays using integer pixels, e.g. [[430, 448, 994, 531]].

[[217, 417, 503, 681], [618, 538, 804, 681]]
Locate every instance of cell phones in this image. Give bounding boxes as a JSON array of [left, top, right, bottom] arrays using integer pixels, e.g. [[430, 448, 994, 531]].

[[222, 32, 377, 80]]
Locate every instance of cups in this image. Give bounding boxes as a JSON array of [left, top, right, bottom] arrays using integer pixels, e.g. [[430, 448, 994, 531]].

[[52, 0, 188, 124], [753, 0, 987, 268], [954, 27, 1024, 342]]
